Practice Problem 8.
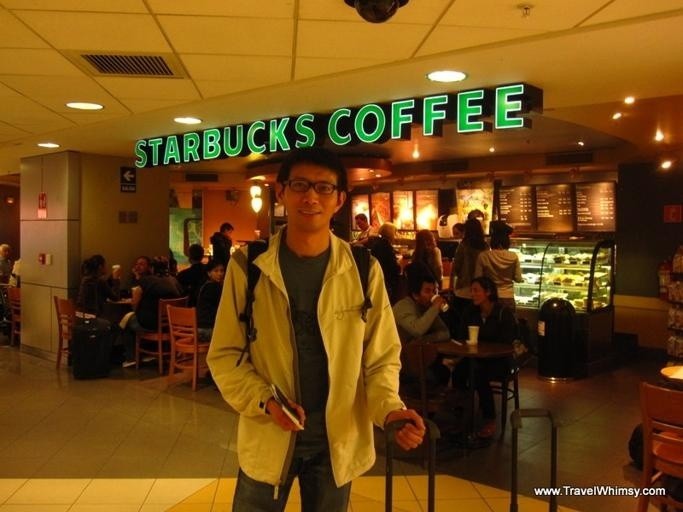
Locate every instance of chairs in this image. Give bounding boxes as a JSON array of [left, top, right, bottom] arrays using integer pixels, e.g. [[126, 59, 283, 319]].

[[0, 271, 215, 392], [400, 318, 682, 512]]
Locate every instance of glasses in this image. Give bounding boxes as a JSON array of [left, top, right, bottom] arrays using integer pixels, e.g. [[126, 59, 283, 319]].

[[278, 178, 342, 195]]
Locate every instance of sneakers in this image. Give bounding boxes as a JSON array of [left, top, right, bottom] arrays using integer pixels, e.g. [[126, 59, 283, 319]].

[[421, 424, 496, 451]]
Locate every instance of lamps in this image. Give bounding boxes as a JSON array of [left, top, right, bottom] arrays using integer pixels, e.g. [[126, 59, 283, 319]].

[[249, 178, 270, 215]]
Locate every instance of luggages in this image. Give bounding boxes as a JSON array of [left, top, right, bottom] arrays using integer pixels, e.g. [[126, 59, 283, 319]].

[[70, 332, 112, 381]]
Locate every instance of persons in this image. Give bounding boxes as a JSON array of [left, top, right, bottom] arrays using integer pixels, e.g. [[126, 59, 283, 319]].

[[204, 148, 426, 512], [0, 243, 15, 286]]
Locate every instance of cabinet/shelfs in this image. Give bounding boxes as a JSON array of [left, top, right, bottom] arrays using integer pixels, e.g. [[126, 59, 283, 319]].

[[512, 238, 617, 349]]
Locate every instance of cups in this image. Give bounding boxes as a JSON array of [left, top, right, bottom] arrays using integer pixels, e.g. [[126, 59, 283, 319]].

[[467, 325, 479, 344], [111, 265, 120, 280], [430, 295, 449, 313]]
